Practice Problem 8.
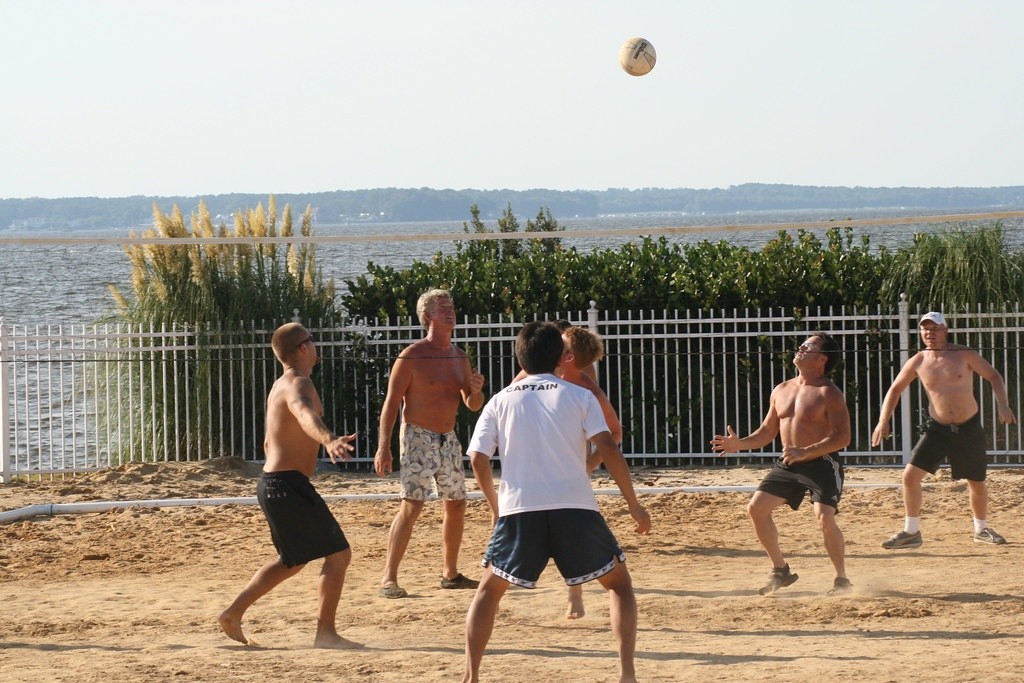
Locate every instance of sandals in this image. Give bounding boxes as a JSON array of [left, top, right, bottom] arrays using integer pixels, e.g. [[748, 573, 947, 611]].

[[759, 562, 799, 596], [826, 577, 854, 596]]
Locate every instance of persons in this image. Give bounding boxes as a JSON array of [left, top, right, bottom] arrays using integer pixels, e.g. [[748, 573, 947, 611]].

[[466, 319, 652, 683], [871, 313, 1016, 550], [220, 321, 364, 648], [711, 334, 851, 596], [374, 290, 485, 599]]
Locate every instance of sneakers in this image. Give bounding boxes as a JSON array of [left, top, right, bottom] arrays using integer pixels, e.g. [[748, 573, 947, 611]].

[[379, 578, 408, 599], [974, 528, 1007, 545], [880, 530, 922, 549], [439, 572, 480, 589]]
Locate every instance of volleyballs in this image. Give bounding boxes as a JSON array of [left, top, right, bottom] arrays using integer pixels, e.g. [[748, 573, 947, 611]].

[[618, 36, 656, 77]]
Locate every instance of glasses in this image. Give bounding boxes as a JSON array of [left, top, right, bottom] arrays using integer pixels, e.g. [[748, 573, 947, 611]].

[[296, 335, 314, 348], [802, 342, 821, 352]]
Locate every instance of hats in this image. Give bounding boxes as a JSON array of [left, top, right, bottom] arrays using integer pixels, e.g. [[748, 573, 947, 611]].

[[918, 312, 947, 329]]
